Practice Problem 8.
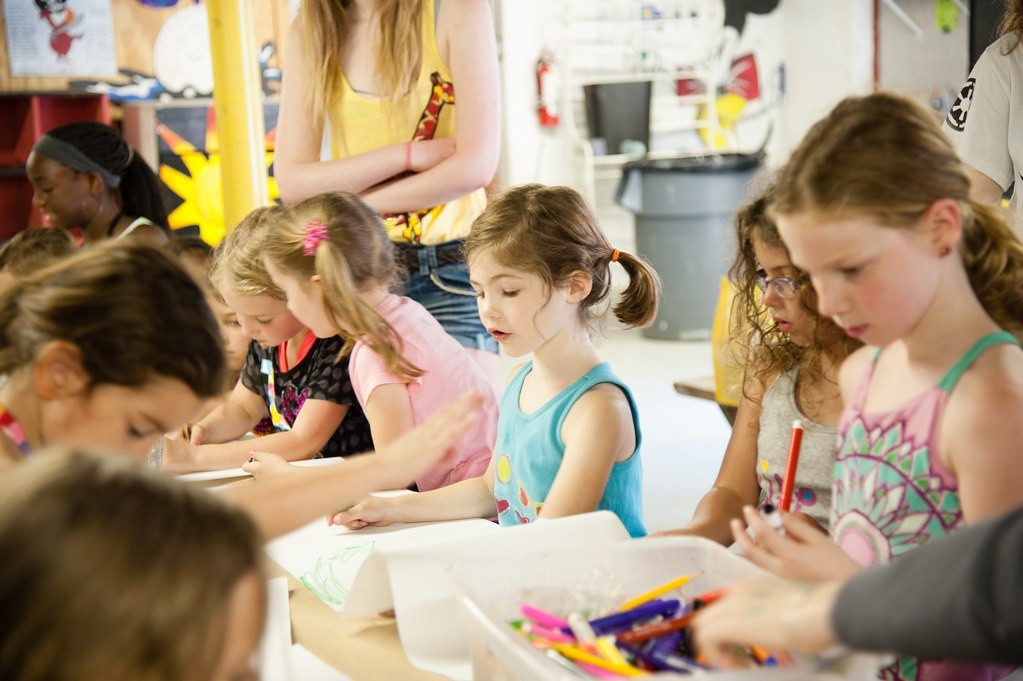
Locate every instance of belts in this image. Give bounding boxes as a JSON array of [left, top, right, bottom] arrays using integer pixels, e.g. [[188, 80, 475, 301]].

[[394, 247, 468, 268]]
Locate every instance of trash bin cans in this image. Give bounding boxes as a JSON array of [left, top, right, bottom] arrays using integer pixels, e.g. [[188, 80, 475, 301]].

[[613, 152, 763, 340]]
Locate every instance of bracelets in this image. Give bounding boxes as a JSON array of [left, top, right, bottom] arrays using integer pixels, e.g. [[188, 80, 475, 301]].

[[404, 141, 412, 173]]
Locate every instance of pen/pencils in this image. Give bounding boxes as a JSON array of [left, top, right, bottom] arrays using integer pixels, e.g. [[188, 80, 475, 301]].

[[778, 419, 806, 513], [183, 424, 190, 443], [509, 570, 798, 680]]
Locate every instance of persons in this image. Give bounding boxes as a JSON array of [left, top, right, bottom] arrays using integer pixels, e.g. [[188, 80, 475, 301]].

[[30, 122, 168, 250], [0, 238, 225, 478], [164, 238, 252, 386], [690, 503, 1023, 671], [275, 1, 501, 359], [942, 0, 1022, 238], [155, 206, 419, 492], [0, 226, 75, 267], [242, 192, 499, 527], [0, 448, 267, 681], [647, 198, 864, 556], [769, 94, 1023, 681], [325, 184, 660, 540]]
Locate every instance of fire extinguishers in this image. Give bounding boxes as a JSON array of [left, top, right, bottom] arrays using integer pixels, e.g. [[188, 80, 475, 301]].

[[536, 50, 559, 127]]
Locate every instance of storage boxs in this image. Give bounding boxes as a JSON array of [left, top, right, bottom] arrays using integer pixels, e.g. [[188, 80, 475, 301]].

[[464, 535, 896, 681]]
